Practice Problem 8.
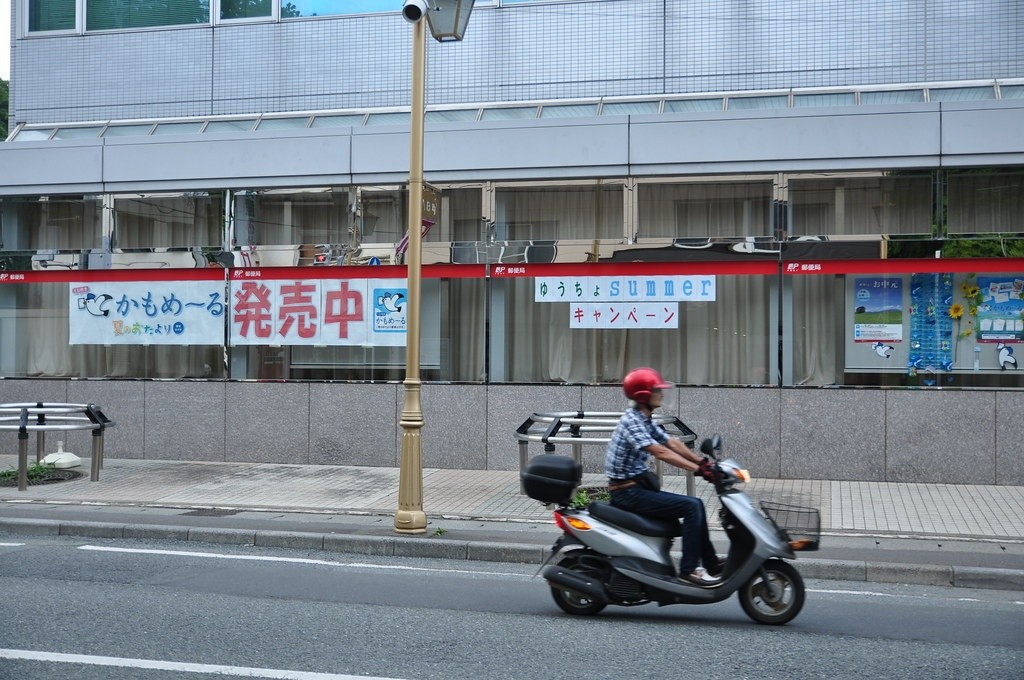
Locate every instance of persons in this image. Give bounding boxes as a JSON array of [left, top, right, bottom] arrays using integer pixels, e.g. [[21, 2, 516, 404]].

[[605, 368, 727, 582]]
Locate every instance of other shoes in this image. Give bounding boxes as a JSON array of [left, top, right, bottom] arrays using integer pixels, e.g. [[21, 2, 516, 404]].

[[705, 553, 730, 576], [676, 567, 724, 589]]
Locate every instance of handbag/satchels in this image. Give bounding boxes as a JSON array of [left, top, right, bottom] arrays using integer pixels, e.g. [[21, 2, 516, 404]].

[[639, 472, 660, 491]]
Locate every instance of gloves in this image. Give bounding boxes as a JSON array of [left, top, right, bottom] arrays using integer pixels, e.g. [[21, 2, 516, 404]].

[[693, 457, 718, 483]]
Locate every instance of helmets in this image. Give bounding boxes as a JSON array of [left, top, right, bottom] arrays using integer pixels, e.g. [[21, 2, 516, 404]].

[[624, 367, 672, 404]]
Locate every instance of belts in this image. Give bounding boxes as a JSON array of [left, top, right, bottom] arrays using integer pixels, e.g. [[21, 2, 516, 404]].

[[608, 478, 638, 490]]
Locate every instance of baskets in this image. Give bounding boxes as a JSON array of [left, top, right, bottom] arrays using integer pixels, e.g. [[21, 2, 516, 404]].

[[760, 499, 821, 552]]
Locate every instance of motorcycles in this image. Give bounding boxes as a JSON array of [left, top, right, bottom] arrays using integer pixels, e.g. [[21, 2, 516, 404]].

[[518, 432, 821, 626]]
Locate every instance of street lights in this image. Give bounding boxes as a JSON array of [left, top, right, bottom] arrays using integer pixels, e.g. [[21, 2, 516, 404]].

[[393, 0, 480, 534]]
